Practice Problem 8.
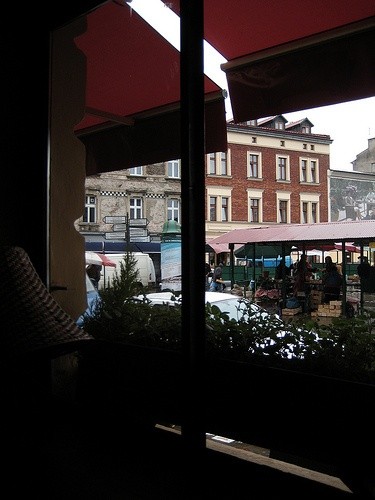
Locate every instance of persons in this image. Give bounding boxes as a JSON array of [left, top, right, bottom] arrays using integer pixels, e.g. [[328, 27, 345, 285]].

[[85, 264, 101, 289], [204, 254, 375, 294]]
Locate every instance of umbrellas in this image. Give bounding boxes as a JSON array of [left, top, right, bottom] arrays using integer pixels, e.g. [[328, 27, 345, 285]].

[[98, 254, 116, 289]]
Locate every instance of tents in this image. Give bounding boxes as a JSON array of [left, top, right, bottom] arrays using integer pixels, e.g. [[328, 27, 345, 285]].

[[85, 252, 103, 265]]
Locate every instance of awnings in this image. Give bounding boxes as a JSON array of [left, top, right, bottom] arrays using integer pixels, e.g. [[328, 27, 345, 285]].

[[53, 0, 375, 177]]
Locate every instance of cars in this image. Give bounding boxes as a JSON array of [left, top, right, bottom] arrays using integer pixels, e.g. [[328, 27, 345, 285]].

[[136, 289, 341, 360]]
